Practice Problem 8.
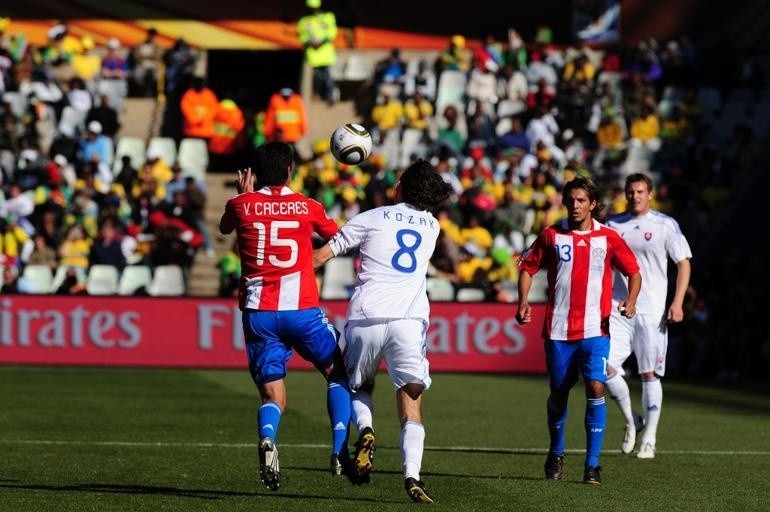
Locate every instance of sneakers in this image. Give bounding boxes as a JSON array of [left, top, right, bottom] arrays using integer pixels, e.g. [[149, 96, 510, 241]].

[[545, 451, 564, 480], [622, 415, 655, 460], [330, 427, 376, 487], [404, 477, 433, 504], [259, 436, 280, 491], [584, 467, 602, 485]]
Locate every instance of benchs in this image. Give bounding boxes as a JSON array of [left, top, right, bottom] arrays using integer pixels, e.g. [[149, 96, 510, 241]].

[[16, 47, 487, 303]]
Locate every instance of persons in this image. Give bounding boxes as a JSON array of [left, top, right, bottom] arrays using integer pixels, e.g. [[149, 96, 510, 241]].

[[219, 140, 352, 491], [311, 158, 453, 506], [603, 172, 693, 460], [515, 176, 643, 485], [1, 1, 770, 300]]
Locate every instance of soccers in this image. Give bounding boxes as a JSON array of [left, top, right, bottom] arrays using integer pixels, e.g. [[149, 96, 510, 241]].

[[332, 123, 373, 164]]
